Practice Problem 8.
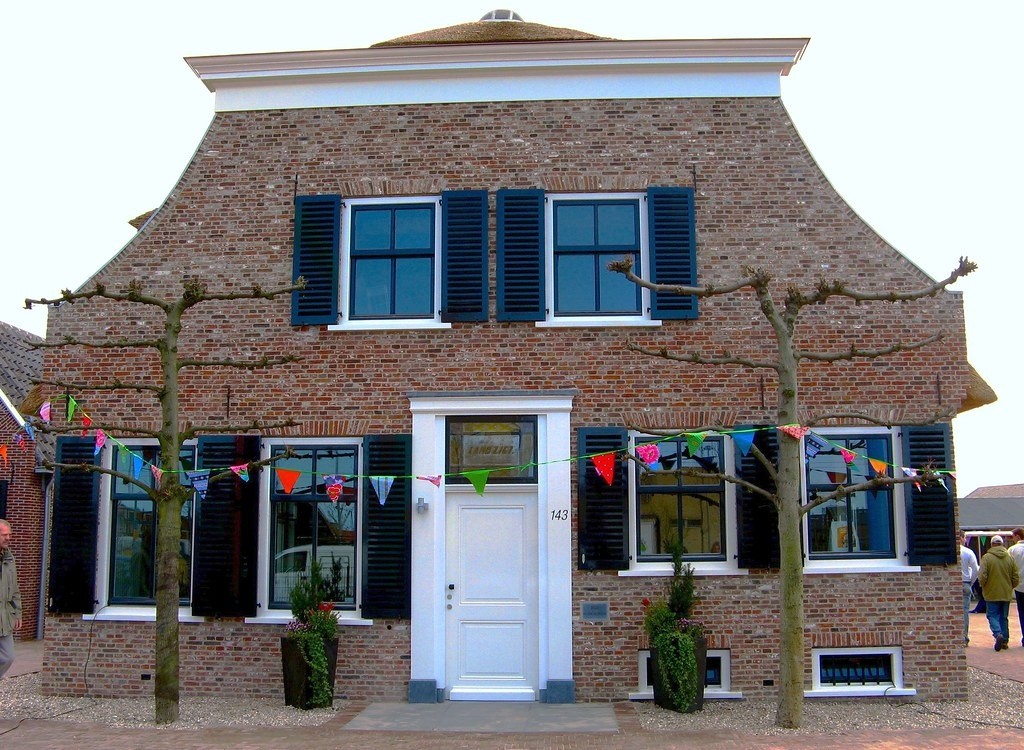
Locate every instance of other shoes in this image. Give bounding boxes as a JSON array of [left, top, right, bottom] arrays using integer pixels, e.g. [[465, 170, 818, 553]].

[[1002, 642, 1008, 649], [995, 635, 1003, 651], [965, 638, 970, 646]]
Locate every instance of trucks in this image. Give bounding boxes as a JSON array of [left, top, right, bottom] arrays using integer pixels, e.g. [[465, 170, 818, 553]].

[[273, 544, 354, 602]]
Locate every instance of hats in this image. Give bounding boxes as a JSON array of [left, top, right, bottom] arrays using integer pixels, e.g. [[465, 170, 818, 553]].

[[990, 535, 1003, 544]]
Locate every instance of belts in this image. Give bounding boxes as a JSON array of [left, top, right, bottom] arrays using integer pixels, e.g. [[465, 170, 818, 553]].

[[963, 581, 970, 584]]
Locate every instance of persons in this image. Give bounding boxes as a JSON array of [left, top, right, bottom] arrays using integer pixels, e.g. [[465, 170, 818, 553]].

[[1008, 527, 1024, 645], [978, 536, 1019, 651], [0, 519, 22, 678], [960, 530, 979, 647]]
